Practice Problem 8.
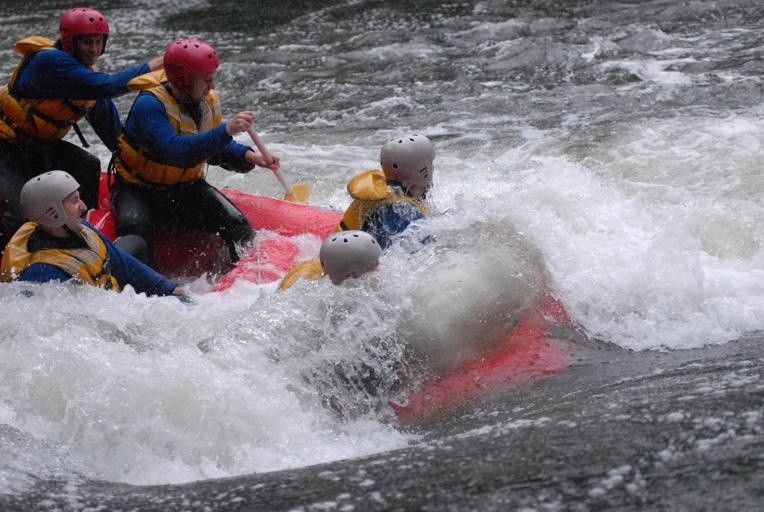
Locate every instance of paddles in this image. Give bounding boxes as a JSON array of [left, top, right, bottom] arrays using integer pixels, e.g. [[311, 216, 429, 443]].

[[248, 122, 308, 204]]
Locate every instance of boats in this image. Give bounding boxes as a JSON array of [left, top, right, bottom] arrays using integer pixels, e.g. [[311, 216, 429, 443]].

[[82, 171, 585, 436]]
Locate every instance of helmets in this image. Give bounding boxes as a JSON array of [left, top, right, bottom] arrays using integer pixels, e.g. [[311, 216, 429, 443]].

[[21, 169, 80, 228], [164, 38, 220, 88], [381, 134, 435, 187], [59, 8, 109, 57]]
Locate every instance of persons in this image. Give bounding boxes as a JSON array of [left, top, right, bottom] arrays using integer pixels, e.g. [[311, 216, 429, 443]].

[[339, 127, 439, 250], [14, 169, 197, 305], [0, 8, 165, 260], [103, 37, 281, 265]]
